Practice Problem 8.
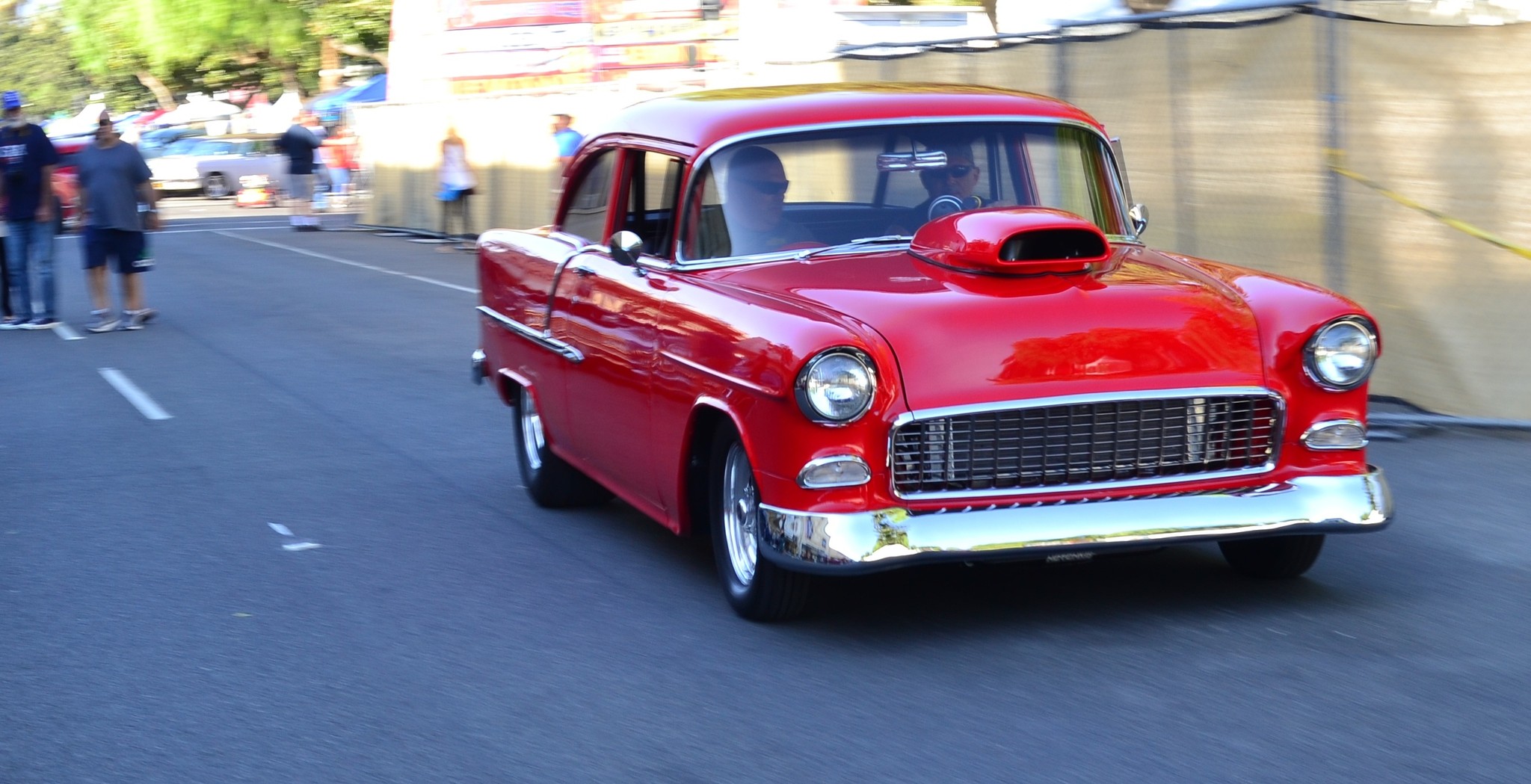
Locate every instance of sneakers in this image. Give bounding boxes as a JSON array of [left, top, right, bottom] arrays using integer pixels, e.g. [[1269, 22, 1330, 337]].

[[18, 315, 65, 330], [1, 315, 33, 330], [120, 308, 160, 331], [85, 309, 123, 333]]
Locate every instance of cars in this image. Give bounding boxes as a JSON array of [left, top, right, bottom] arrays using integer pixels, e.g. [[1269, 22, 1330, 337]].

[[38, 73, 387, 217], [469, 79, 1395, 627]]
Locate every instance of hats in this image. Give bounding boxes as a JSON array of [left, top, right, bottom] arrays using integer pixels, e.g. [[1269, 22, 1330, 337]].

[[925, 137, 974, 162], [3, 90, 22, 109]]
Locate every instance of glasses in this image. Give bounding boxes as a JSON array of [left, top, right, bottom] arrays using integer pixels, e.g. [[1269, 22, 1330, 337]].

[[729, 176, 790, 194], [99, 119, 111, 126], [920, 165, 974, 179]]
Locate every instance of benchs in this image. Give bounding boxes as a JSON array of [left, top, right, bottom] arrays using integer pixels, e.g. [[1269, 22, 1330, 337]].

[[621, 205, 912, 263]]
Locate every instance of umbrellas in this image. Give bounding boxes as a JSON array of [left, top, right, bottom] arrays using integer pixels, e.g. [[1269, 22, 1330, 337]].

[[42, 70, 395, 153]]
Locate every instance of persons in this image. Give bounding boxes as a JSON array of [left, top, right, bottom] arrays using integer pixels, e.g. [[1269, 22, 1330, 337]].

[[1, 219, 21, 320], [274, 113, 324, 234], [1, 89, 64, 332], [74, 107, 162, 332], [551, 110, 586, 177], [881, 139, 1018, 240], [434, 122, 477, 241], [691, 144, 814, 261]]
